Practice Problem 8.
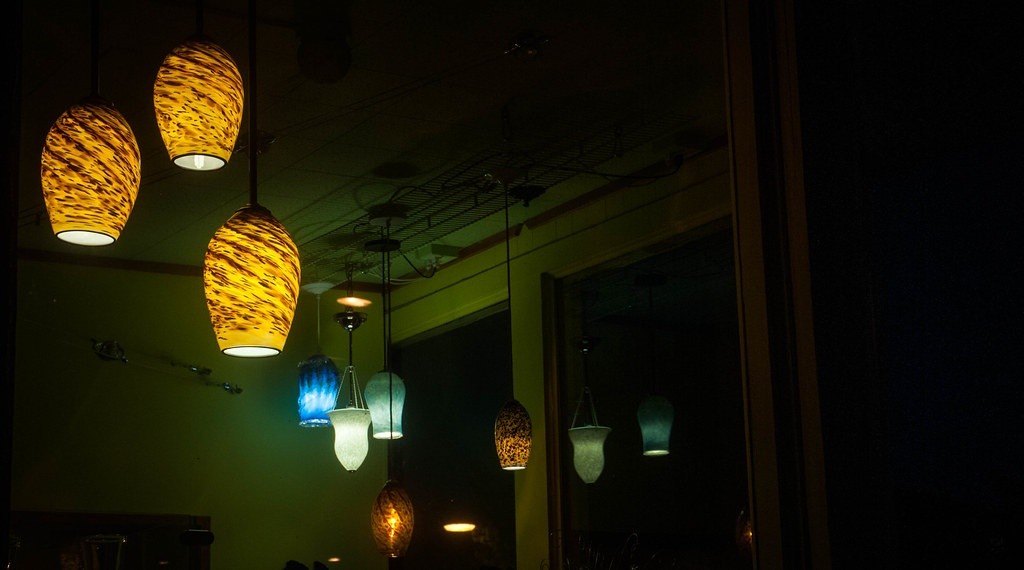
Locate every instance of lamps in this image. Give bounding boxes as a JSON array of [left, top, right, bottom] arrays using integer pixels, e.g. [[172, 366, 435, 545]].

[[40, 1, 613, 562], [635, 390, 677, 459]]
